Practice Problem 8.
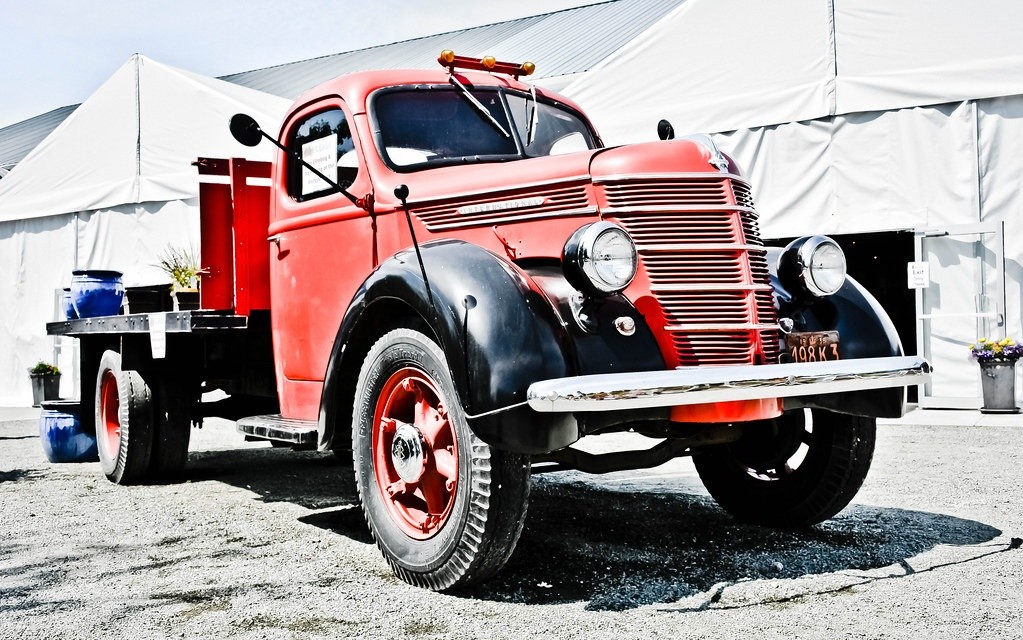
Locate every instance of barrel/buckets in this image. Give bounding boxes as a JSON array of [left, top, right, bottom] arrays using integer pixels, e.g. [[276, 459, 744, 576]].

[[124, 284, 174, 313]]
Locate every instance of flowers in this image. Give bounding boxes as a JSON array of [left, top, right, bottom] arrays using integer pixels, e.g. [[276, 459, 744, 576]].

[[973, 339, 1023, 362]]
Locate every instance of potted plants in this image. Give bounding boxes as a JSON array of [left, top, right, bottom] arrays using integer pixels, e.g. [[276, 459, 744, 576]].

[[31, 364, 61, 407], [166, 246, 210, 311]]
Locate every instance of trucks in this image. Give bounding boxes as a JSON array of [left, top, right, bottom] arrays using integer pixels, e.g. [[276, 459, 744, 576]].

[[45, 49, 935, 596]]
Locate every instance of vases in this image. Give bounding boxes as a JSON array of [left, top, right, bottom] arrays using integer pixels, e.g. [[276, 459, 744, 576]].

[[980, 361, 1014, 410], [126, 284, 174, 314], [69, 269, 125, 316], [37, 399, 99, 463], [65, 288, 79, 319]]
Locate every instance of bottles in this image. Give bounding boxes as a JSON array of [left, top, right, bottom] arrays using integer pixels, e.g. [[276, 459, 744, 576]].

[[63, 287, 76, 320], [70, 269, 126, 321], [39, 400, 98, 463]]
